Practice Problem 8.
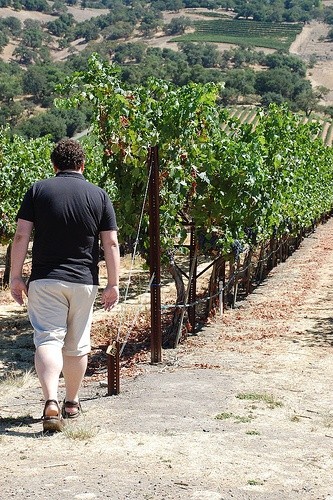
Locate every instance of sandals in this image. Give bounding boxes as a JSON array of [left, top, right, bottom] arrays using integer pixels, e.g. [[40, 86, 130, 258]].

[[41, 400, 64, 431], [62, 397, 81, 419]]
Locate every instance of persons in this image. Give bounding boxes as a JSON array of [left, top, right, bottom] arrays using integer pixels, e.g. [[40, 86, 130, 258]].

[[8, 141, 120, 430]]
